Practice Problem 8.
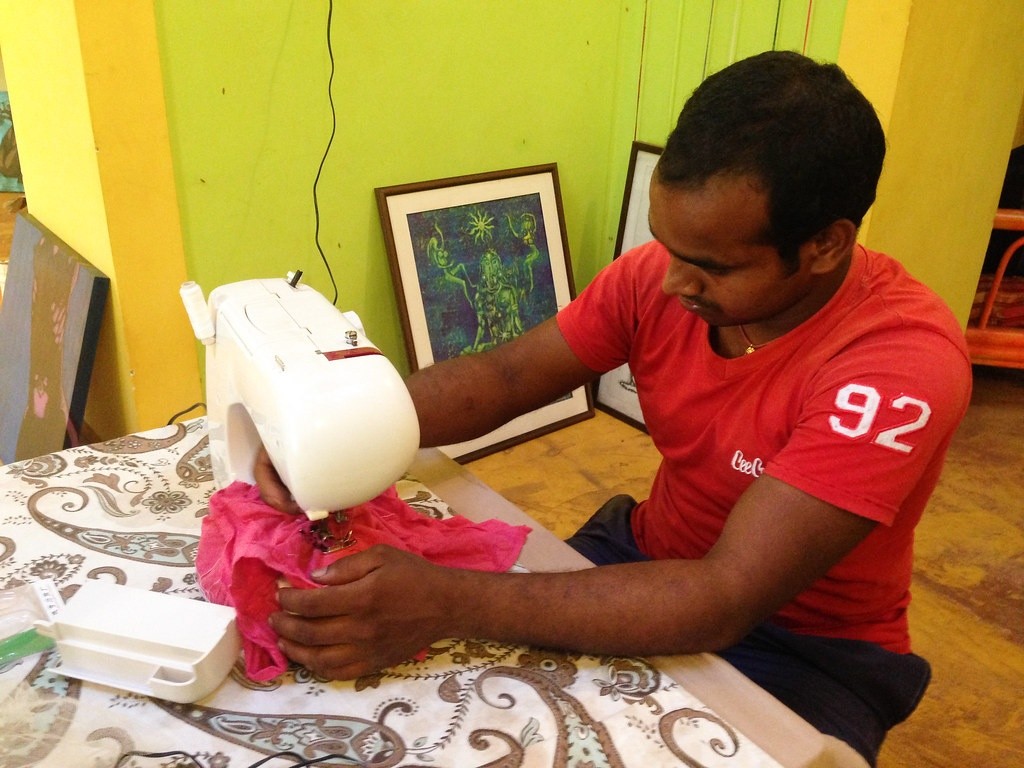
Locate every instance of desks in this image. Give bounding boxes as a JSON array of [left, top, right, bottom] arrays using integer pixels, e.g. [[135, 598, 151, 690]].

[[0, 401, 870, 768]]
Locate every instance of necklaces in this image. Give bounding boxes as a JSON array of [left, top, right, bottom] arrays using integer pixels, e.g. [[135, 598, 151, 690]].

[[738, 324, 767, 355]]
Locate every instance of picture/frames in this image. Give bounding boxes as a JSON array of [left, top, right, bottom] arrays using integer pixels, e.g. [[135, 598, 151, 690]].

[[598, 139, 663, 432], [374, 162, 592, 467]]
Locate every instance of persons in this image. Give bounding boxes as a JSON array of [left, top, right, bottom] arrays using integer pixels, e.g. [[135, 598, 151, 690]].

[[266, 50, 973, 767]]
